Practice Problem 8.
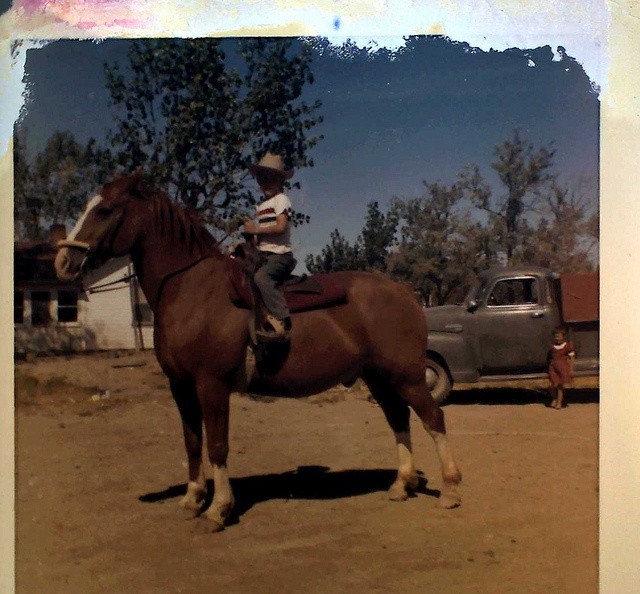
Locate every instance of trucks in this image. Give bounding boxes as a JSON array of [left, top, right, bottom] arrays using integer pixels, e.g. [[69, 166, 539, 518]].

[[415, 269, 597, 405]]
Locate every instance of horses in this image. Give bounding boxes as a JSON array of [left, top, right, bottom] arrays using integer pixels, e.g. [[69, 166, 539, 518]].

[[53, 162, 460, 537]]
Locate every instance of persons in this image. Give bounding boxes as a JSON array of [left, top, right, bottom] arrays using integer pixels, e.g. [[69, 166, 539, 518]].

[[545, 327, 576, 411], [242, 152, 298, 335]]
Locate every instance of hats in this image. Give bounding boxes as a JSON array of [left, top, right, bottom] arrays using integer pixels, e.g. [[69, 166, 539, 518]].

[[246, 151, 294, 180]]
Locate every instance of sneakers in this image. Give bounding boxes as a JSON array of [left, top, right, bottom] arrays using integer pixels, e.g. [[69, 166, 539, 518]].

[[258, 316, 292, 337]]
